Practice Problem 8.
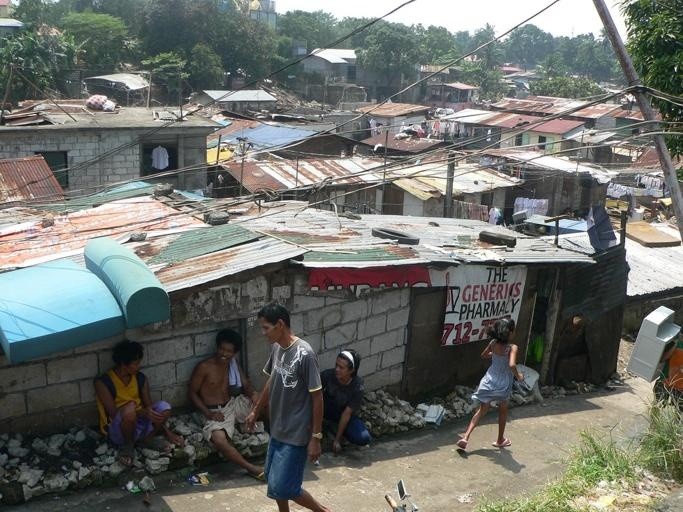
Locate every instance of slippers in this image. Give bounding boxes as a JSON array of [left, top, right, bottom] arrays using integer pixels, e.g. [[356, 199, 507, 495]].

[[135, 438, 171, 452], [119, 448, 134, 466], [249, 469, 265, 481], [493, 439, 511, 447], [456, 439, 467, 449]]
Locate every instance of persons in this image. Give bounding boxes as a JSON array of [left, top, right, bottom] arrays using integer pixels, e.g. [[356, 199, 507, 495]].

[[457, 319, 523, 450], [320, 349, 373, 457], [188, 330, 268, 481], [244, 303, 331, 511], [92, 339, 186, 466], [627, 201, 635, 218]]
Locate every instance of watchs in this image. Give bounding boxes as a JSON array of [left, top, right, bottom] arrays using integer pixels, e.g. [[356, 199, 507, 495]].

[[311, 432, 323, 440]]
